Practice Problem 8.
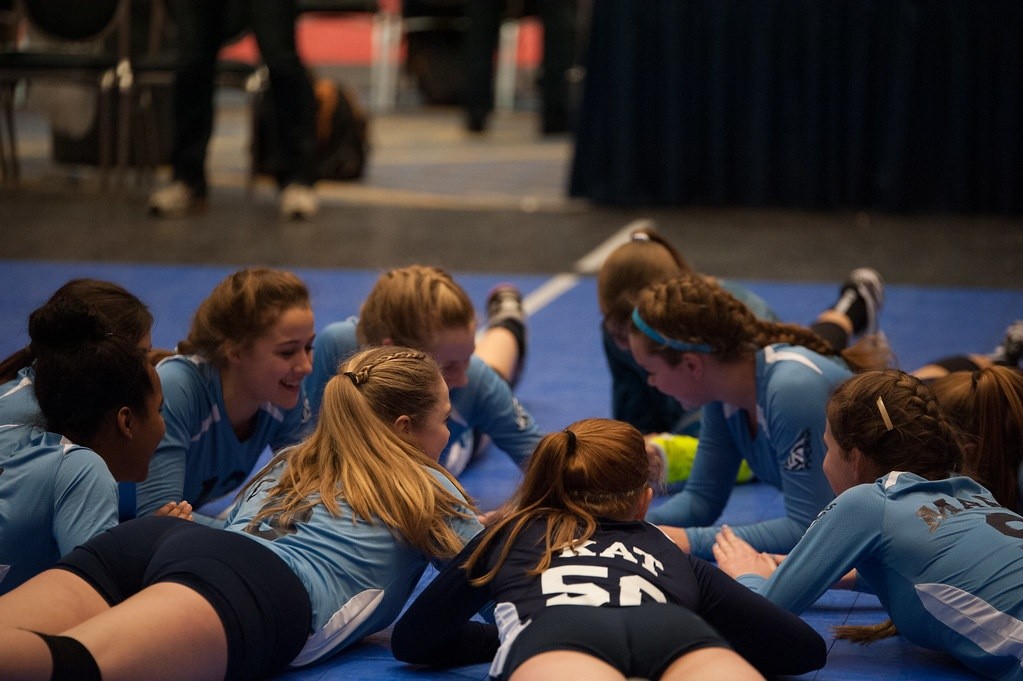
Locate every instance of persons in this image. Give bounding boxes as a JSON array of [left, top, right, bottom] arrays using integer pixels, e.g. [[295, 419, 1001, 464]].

[[304, 264, 546, 480], [391, 418, 827, 681], [0, 279, 193, 597], [148, 0, 323, 221], [313, 75, 368, 183], [0, 346, 495, 681], [597, 227, 1023, 681], [119, 267, 313, 530]]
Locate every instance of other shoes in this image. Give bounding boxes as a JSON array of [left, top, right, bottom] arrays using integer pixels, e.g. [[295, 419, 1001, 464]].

[[282, 183, 315, 219], [150, 183, 201, 216], [485, 284, 525, 350], [834, 264, 882, 339]]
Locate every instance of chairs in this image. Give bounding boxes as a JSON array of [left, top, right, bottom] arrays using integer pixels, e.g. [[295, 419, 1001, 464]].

[[0, 0, 263, 222]]
